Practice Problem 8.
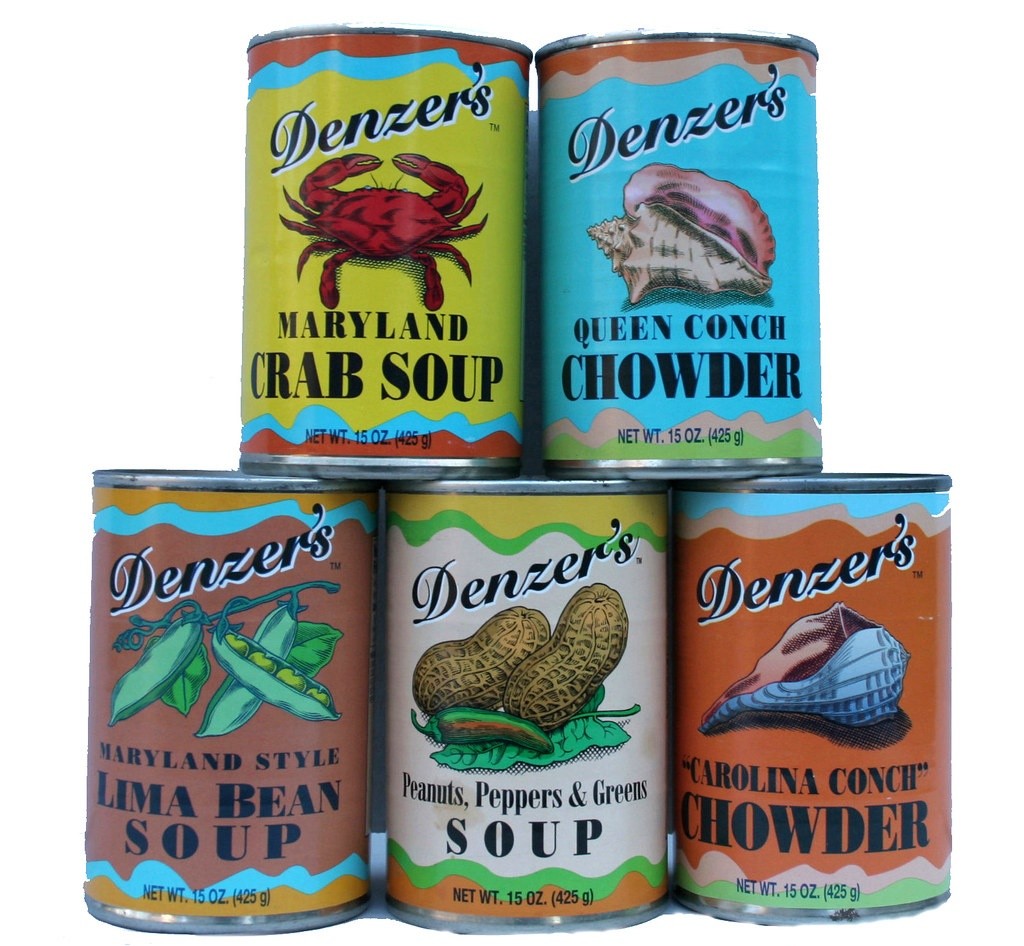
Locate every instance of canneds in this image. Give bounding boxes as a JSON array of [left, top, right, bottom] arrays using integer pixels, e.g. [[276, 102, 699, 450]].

[[81, 26, 955, 938]]
